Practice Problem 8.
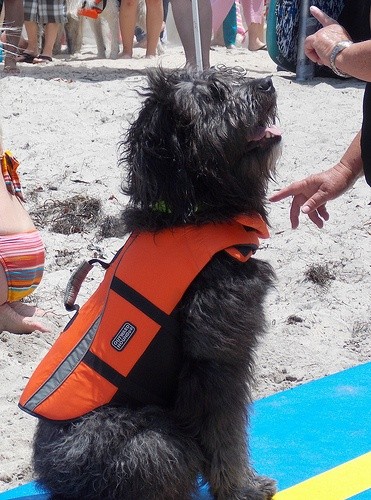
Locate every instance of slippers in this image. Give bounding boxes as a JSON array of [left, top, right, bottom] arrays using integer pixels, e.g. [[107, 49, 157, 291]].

[[249, 45, 268, 52], [16, 52, 56, 65]]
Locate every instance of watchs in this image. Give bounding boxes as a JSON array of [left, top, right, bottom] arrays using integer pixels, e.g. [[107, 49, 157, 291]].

[[328, 41, 354, 78]]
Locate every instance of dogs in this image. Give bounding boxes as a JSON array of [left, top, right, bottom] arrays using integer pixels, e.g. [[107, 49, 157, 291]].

[[30, 65, 285, 500]]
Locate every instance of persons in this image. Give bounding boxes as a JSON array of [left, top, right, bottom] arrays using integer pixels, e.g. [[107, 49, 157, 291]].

[[0, 0, 273, 73], [267, 5, 370, 229], [0, 145, 46, 334]]
